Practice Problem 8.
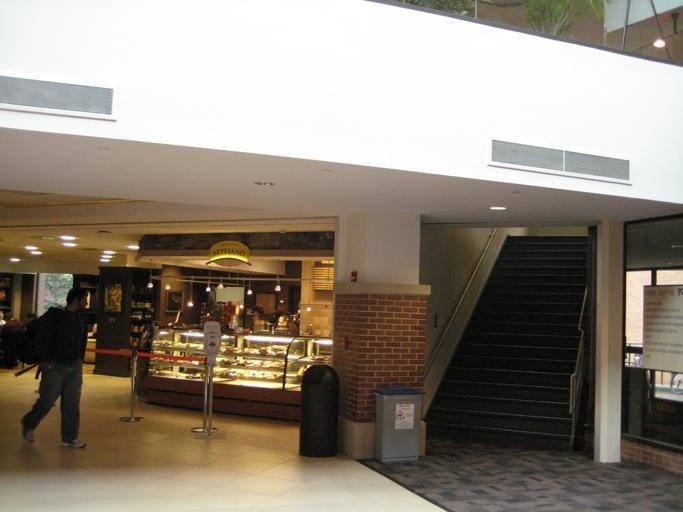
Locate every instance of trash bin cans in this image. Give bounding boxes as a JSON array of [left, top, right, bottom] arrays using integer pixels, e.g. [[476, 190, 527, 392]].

[[374, 387, 426, 463], [300, 364, 339, 458]]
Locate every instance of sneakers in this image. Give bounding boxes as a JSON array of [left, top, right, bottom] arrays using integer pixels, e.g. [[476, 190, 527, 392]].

[[61, 437, 87, 448], [21, 415, 34, 442]]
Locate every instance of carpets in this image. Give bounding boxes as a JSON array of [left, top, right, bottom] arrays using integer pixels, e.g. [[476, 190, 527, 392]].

[[355, 439, 683, 512]]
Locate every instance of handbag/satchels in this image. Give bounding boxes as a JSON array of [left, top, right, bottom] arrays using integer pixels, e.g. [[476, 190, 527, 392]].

[[22, 307, 65, 362]]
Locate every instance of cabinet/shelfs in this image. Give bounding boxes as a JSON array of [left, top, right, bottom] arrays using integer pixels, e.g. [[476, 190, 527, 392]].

[[149, 325, 332, 392]]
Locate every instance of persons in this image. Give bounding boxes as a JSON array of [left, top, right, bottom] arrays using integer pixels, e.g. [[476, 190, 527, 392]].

[[25, 312, 39, 330], [244, 306, 270, 334], [22, 286, 89, 448], [1, 313, 23, 334], [0, 311, 7, 325]]
